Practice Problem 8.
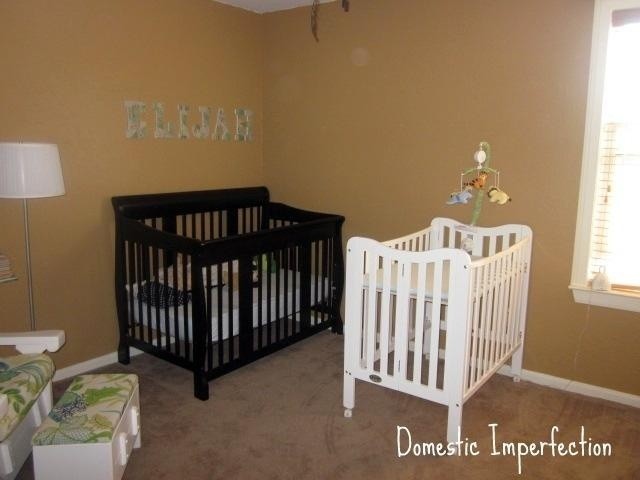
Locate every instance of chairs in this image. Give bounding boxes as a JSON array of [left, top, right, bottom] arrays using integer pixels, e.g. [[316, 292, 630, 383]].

[[1, 328, 145, 479]]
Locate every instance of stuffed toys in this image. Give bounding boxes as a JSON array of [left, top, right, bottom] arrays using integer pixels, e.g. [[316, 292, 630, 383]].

[[445, 140, 512, 223]]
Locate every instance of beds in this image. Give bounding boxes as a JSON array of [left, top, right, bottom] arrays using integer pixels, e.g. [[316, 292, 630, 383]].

[[107, 185, 346, 402], [342, 216, 533, 454]]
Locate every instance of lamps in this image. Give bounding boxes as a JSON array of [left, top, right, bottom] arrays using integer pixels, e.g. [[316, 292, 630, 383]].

[[0, 139, 68, 328]]
